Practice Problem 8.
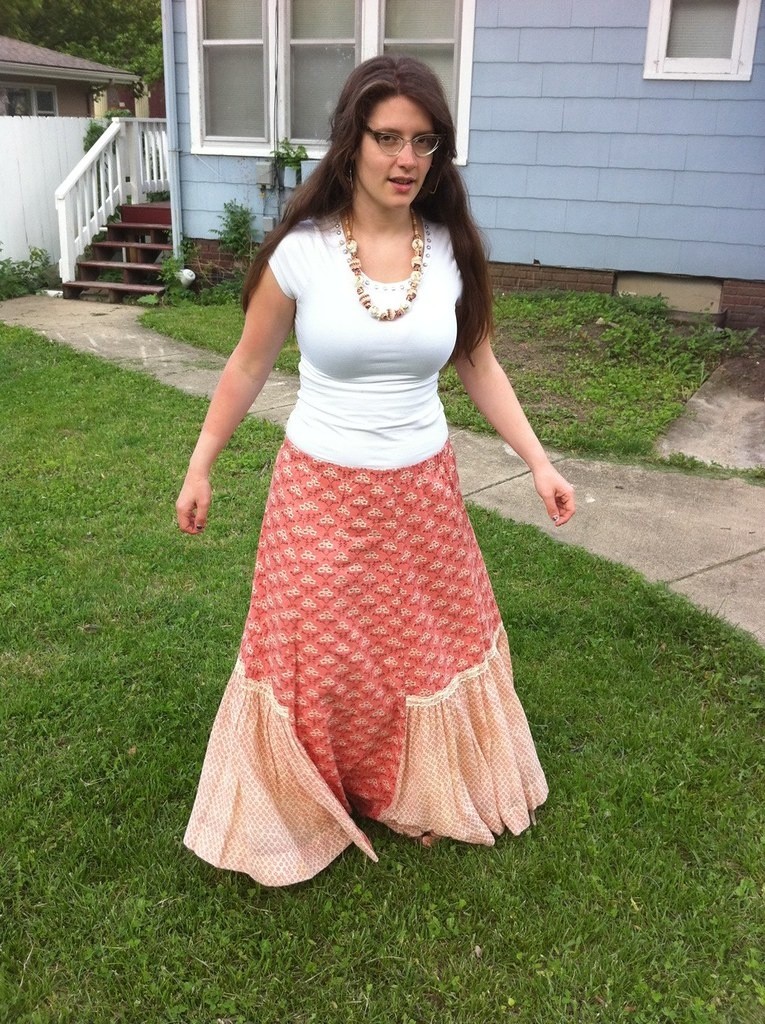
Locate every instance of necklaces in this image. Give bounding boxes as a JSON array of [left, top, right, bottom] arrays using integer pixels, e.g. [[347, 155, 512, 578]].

[[345, 209, 424, 320]]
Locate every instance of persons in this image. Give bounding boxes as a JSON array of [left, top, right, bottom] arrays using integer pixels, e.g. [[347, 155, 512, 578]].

[[176, 56, 576, 886]]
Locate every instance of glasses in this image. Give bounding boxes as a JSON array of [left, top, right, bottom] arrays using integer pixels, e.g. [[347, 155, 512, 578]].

[[363, 124, 446, 157]]
